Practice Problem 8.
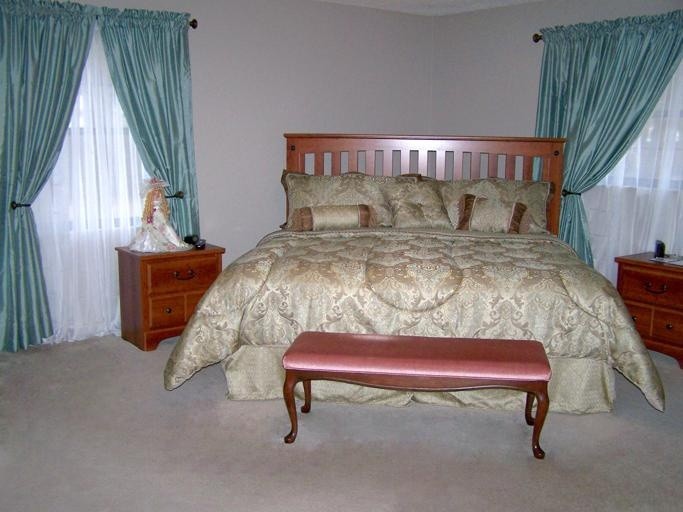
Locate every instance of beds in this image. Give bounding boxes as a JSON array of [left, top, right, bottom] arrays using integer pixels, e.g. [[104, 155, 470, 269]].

[[224, 131, 610, 412]]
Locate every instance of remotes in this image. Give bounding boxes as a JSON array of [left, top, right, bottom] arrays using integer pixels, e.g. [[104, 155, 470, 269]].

[[196, 239, 206, 249]]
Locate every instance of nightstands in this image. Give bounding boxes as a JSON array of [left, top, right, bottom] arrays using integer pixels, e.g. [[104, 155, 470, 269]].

[[613, 252, 683, 369], [114, 244, 225, 351]]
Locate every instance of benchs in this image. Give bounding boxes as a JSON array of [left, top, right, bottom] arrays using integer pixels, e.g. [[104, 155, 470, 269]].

[[281, 331, 553, 460]]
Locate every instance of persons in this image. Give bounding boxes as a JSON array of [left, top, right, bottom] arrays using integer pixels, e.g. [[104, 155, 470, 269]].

[[130, 176, 197, 253]]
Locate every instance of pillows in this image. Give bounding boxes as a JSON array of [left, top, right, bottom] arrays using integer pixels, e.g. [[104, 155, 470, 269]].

[[278, 168, 556, 237]]
